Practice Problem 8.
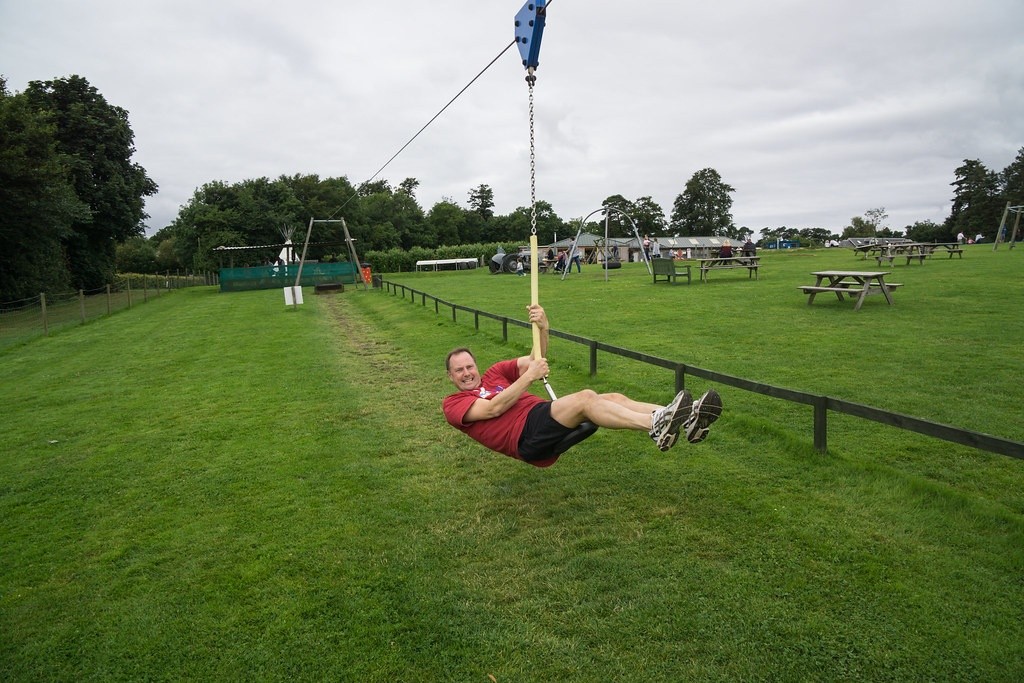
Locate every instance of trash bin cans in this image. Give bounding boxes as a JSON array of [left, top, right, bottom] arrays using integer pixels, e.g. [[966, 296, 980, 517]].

[[360, 263, 371, 283]]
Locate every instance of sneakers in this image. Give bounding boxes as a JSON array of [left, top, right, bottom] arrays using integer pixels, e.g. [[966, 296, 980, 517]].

[[682, 389, 722, 443], [648, 389, 694, 453]]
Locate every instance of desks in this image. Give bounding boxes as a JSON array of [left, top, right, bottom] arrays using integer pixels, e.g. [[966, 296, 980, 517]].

[[542, 259, 557, 272], [928, 243, 963, 259], [808, 270, 894, 310], [877, 243, 925, 268], [697, 256, 761, 282]]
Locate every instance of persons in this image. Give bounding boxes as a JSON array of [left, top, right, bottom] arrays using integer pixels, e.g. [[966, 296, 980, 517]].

[[441, 304, 723, 468], [719, 240, 732, 257], [825, 240, 839, 248], [517, 258, 527, 277], [547, 234, 661, 274], [743, 239, 756, 256], [957, 230, 985, 244]]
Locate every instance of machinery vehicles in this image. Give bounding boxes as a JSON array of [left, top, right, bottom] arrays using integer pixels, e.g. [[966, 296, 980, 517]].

[[488, 243, 548, 275]]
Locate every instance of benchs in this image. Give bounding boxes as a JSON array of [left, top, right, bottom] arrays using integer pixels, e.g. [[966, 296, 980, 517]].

[[836, 281, 904, 292], [696, 264, 762, 269], [871, 248, 964, 259], [796, 285, 864, 297], [651, 255, 692, 285]]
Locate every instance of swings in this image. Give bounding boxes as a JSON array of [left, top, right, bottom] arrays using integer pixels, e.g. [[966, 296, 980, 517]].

[[602, 210, 622, 270], [1009, 211, 1016, 247]]
